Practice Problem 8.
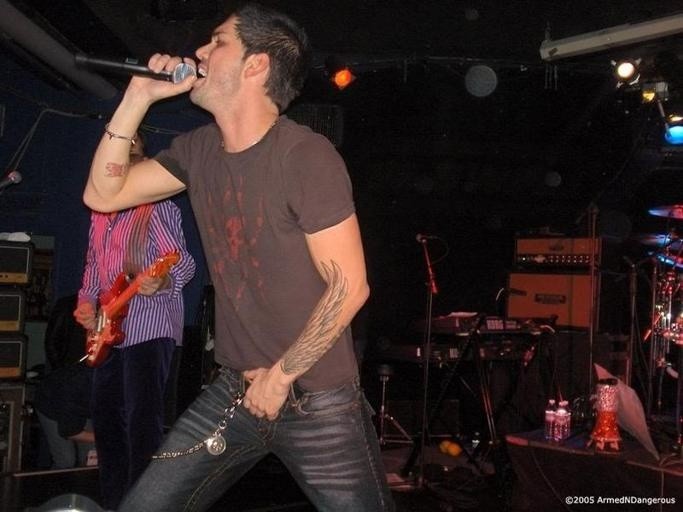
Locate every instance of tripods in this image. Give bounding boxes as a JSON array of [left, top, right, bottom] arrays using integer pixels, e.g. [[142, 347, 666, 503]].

[[634, 271, 683, 455], [387, 248, 468, 512], [371, 360, 413, 446]]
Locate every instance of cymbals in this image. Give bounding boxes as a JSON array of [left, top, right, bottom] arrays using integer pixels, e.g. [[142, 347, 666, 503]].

[[632, 233, 683, 249], [649, 238, 683, 269], [648, 204, 683, 219]]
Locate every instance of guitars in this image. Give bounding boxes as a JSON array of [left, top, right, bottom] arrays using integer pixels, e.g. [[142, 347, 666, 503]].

[[85, 251, 181, 367]]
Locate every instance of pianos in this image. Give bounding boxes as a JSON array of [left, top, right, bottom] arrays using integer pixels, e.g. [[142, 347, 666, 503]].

[[367, 317, 555, 366]]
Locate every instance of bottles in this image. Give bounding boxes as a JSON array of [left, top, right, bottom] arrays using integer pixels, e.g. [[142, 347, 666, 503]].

[[544, 399, 572, 442]]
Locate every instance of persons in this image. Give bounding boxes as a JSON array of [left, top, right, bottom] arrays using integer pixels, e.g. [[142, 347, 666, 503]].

[[30, 294, 114, 481], [77, 4, 400, 510], [71, 111, 196, 511]]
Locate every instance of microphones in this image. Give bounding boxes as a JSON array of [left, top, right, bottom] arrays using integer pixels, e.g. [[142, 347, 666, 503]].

[[414, 232, 438, 244], [1, 170, 23, 188], [75, 48, 196, 87]]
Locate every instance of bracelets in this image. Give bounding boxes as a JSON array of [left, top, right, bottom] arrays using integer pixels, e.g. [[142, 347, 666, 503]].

[[102, 122, 139, 146]]
